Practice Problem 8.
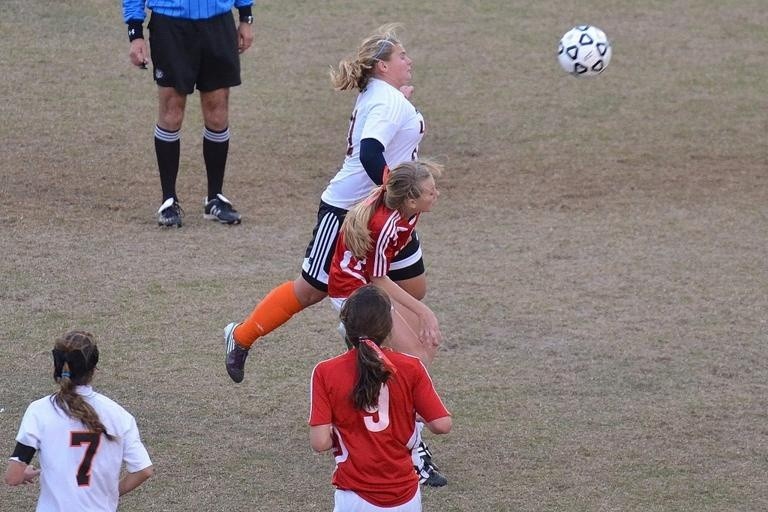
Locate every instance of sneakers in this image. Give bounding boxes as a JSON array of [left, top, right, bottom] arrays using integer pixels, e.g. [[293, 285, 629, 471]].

[[156, 197, 184, 228], [412, 436, 448, 488], [201, 193, 243, 227], [221, 319, 249, 384]]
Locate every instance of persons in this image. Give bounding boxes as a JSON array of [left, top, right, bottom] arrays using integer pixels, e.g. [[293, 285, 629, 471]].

[[2, 328, 156, 511], [121, 0, 257, 229], [222, 24, 428, 385], [325, 159, 450, 488], [306, 283, 454, 512]]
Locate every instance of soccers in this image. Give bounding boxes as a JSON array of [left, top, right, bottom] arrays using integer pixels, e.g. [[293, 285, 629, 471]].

[[558, 25, 611, 78]]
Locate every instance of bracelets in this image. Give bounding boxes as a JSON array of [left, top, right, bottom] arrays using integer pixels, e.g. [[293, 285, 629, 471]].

[[239, 14, 254, 26], [127, 22, 145, 42]]
